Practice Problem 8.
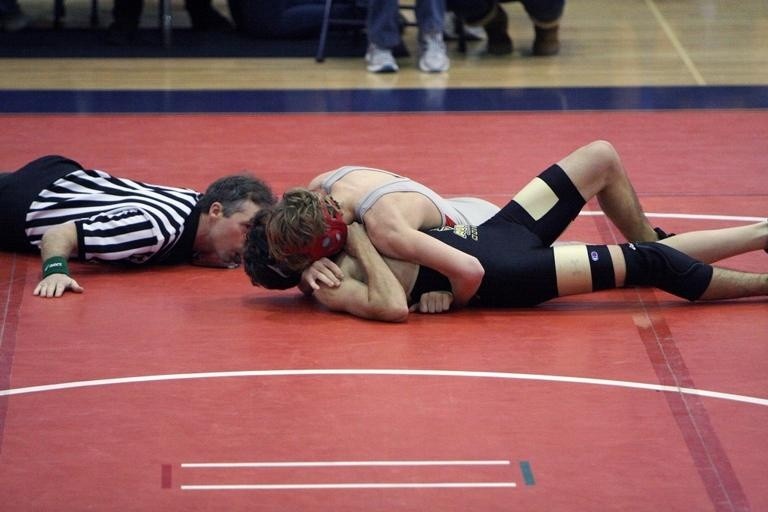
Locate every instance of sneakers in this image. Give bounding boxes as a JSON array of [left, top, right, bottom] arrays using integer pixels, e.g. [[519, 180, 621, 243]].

[[419, 33, 451, 72], [364, 43, 399, 73]]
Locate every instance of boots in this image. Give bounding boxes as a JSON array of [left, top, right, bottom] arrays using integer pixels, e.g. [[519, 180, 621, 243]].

[[482, 5, 514, 52], [532, 23, 562, 53]]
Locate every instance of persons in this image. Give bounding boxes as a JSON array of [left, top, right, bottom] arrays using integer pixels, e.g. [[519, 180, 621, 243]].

[[273, 162, 767, 317], [364, 0, 451, 74], [238, 135, 767, 320], [449, 0, 567, 56], [1, 155, 276, 299], [108, 0, 230, 37]]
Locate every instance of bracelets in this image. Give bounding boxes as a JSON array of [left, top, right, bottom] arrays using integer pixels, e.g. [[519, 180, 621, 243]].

[[39, 254, 71, 275]]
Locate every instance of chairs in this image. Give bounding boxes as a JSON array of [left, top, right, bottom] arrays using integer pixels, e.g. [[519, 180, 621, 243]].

[[54, 0, 467, 64]]
